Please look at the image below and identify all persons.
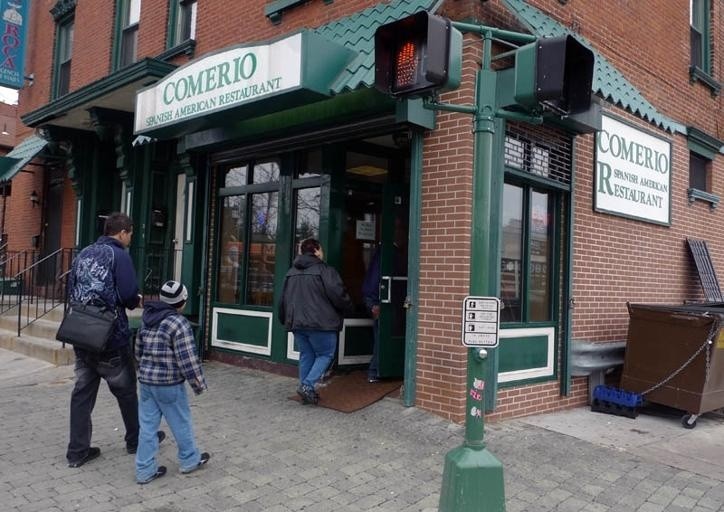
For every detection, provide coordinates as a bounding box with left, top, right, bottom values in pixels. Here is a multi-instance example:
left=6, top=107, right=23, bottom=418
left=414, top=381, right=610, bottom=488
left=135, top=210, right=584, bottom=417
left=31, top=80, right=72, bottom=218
left=133, top=279, right=212, bottom=483
left=360, top=225, right=381, bottom=384
left=63, top=212, right=166, bottom=468
left=276, top=236, right=356, bottom=406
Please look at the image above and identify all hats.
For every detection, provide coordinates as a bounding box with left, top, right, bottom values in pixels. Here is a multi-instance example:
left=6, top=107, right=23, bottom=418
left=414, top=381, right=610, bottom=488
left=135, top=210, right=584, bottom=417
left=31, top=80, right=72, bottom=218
left=160, top=280, right=189, bottom=309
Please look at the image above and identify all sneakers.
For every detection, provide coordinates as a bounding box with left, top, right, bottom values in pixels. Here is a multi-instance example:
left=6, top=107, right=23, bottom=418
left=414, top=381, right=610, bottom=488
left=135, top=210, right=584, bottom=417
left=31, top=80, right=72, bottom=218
left=67, top=447, right=100, bottom=467
left=137, top=466, right=165, bottom=484
left=296, top=383, right=320, bottom=405
left=128, top=430, right=165, bottom=453
left=368, top=376, right=382, bottom=383
left=182, top=452, right=210, bottom=474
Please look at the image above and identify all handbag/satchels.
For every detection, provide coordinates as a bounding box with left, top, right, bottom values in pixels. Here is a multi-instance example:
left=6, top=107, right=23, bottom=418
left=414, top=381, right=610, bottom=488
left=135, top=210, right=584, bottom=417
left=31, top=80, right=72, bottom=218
left=56, top=302, right=117, bottom=355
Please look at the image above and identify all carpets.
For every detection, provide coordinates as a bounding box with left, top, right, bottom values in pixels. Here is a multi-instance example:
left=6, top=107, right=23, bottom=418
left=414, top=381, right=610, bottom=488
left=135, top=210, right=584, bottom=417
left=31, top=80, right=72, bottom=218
left=289, top=370, right=404, bottom=414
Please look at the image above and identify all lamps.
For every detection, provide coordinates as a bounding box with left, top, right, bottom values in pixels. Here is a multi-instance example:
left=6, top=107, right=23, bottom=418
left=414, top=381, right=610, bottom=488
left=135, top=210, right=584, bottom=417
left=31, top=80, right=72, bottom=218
left=30, top=190, right=39, bottom=208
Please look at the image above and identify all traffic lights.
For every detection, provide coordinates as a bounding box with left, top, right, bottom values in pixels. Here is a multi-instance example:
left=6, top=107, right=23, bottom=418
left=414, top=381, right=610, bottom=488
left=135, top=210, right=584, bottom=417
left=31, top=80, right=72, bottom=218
left=371, top=10, right=451, bottom=100
left=535, top=30, right=597, bottom=115
